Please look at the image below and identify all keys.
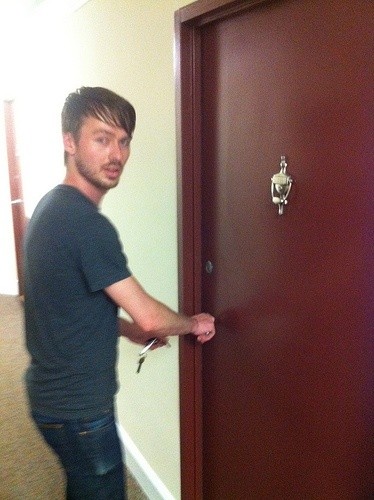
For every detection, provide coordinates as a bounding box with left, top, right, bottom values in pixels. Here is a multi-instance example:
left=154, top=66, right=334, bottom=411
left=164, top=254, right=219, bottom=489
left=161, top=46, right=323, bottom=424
left=135, top=351, right=148, bottom=374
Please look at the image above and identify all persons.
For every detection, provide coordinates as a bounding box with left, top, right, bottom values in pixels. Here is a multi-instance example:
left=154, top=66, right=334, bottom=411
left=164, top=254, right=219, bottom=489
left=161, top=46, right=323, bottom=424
left=22, top=86, right=216, bottom=499
